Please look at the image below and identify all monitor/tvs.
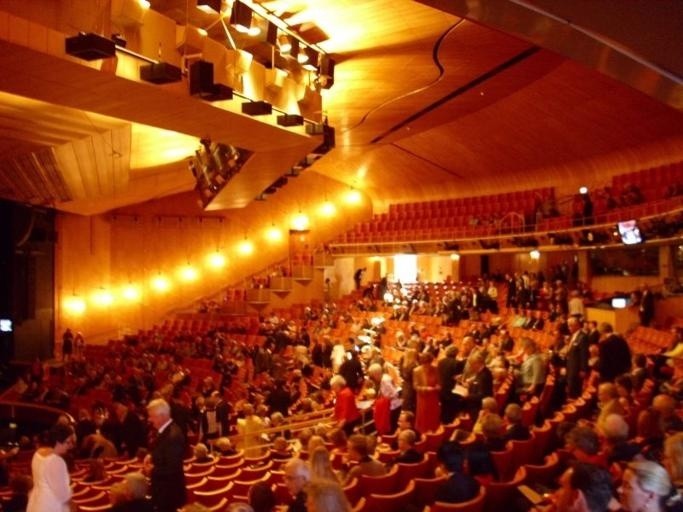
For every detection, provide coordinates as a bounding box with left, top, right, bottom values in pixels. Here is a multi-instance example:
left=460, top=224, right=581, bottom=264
left=617, top=219, right=644, bottom=246
left=611, top=298, right=626, bottom=309
left=0, top=319, right=14, bottom=333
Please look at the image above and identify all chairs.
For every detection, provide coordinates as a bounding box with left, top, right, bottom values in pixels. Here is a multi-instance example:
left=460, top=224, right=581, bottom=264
left=0, top=157, right=683, bottom=512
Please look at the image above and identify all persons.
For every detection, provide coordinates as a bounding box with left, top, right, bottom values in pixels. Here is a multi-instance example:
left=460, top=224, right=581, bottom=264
left=1, top=180, right=682, bottom=512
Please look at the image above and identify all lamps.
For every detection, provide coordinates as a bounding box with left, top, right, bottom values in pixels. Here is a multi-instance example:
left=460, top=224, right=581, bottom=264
left=193, top=0, right=337, bottom=91
left=61, top=33, right=332, bottom=143
left=254, top=123, right=336, bottom=205
left=181, top=136, right=243, bottom=199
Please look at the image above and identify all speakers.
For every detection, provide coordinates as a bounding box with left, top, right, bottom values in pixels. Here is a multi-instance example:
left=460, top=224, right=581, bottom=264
left=189, top=61, right=214, bottom=98
